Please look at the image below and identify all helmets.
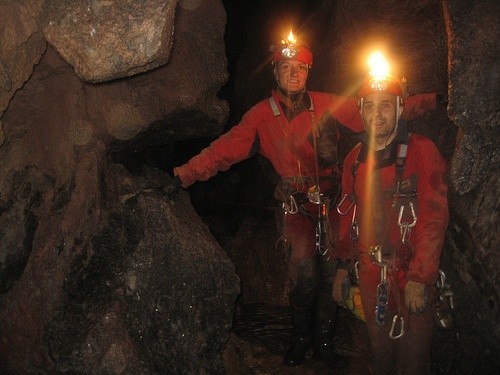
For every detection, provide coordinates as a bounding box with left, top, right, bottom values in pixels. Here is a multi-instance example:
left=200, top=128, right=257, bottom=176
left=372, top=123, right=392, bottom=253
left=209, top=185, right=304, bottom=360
left=357, top=75, right=403, bottom=100
left=273, top=40, right=313, bottom=68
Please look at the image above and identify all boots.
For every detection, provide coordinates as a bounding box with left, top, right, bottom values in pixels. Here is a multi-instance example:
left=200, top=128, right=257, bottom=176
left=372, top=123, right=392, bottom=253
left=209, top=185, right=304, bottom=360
left=282, top=288, right=312, bottom=367
left=314, top=283, right=340, bottom=374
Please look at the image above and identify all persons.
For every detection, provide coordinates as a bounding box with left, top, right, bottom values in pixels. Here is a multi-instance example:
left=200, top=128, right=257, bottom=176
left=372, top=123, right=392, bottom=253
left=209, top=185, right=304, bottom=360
left=332, top=79, right=449, bottom=373
left=147, top=42, right=446, bottom=368
